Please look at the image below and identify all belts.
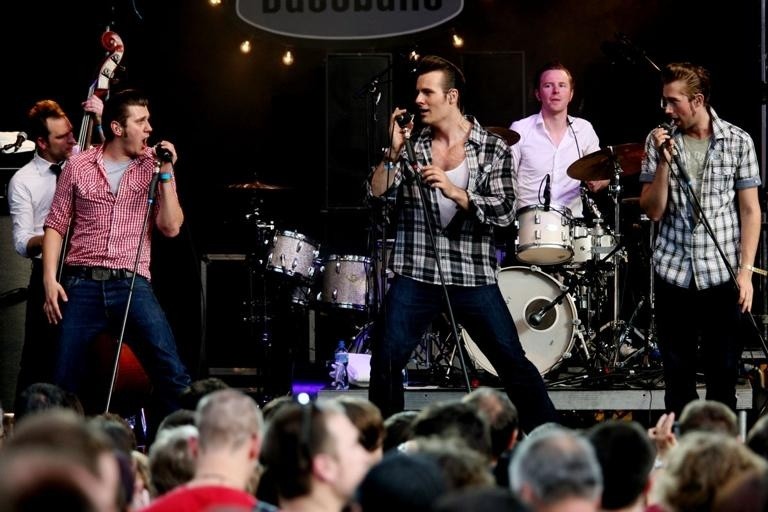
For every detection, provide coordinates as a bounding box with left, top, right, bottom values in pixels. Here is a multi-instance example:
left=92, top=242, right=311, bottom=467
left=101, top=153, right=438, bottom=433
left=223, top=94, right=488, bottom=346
left=65, top=266, right=133, bottom=281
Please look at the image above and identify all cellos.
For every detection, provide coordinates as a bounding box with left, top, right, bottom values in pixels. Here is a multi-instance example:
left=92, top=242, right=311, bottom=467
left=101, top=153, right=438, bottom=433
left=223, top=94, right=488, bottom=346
left=77, top=26, right=150, bottom=397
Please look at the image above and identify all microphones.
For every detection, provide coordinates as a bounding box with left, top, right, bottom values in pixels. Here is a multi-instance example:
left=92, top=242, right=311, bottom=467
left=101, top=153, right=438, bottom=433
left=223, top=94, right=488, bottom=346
left=14, top=131, right=27, bottom=152
left=152, top=142, right=172, bottom=162
left=657, top=118, right=681, bottom=152
left=395, top=112, right=411, bottom=128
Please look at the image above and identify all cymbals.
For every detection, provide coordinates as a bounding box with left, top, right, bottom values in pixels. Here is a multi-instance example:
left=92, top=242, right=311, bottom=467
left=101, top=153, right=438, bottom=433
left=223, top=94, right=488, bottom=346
left=567, top=142, right=648, bottom=181
left=622, top=197, right=644, bottom=203
left=226, top=181, right=281, bottom=192
left=317, top=205, right=371, bottom=211
left=483, top=126, right=520, bottom=147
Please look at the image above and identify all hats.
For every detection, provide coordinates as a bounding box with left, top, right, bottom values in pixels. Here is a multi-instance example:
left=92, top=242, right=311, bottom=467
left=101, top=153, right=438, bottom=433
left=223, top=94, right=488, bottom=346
left=423, top=45, right=466, bottom=83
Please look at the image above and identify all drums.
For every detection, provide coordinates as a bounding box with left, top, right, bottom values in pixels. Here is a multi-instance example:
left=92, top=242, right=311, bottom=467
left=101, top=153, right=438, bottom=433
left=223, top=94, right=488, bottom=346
left=515, top=205, right=575, bottom=265
left=316, top=255, right=377, bottom=312
left=292, top=286, right=311, bottom=305
left=265, top=230, right=322, bottom=281
left=461, top=267, right=581, bottom=377
left=562, top=227, right=618, bottom=277
left=377, top=238, right=397, bottom=277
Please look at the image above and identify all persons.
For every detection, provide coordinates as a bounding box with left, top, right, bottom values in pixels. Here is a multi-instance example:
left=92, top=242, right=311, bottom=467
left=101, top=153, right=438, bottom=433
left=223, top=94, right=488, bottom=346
left=178, top=379, right=229, bottom=412
left=379, top=411, right=422, bottom=457
left=146, top=426, right=202, bottom=500
left=9, top=95, right=107, bottom=430
left=40, top=87, right=190, bottom=414
left=591, top=420, right=654, bottom=512
left=409, top=398, right=498, bottom=473
left=140, top=388, right=280, bottom=512
left=675, top=400, right=742, bottom=449
left=0, top=406, right=120, bottom=511
left=509, top=65, right=609, bottom=221
left=509, top=423, right=603, bottom=512
left=463, top=388, right=519, bottom=468
left=10, top=381, right=84, bottom=434
left=641, top=62, right=763, bottom=424
left=261, top=399, right=367, bottom=512
left=333, top=395, right=384, bottom=465
left=365, top=57, right=562, bottom=439
left=353, top=438, right=497, bottom=512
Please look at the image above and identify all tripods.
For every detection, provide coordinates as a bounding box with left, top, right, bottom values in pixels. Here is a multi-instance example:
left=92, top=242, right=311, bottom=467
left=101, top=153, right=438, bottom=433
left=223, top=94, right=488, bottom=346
left=421, top=316, right=471, bottom=387
left=578, top=185, right=661, bottom=387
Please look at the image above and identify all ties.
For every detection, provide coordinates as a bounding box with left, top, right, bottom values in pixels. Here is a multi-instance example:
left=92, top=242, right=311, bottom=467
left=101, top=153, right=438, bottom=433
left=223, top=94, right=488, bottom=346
left=49, top=162, right=63, bottom=186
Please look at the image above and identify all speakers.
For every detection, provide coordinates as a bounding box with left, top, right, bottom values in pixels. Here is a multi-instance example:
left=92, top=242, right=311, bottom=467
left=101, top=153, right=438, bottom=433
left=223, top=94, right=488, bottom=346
left=623, top=198, right=660, bottom=268
